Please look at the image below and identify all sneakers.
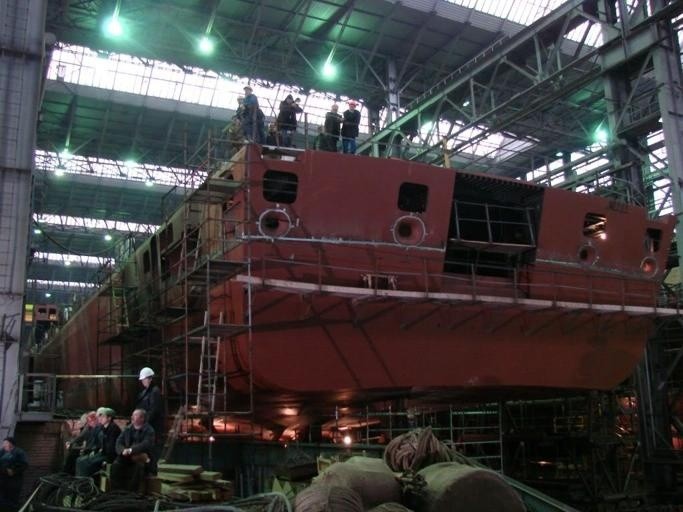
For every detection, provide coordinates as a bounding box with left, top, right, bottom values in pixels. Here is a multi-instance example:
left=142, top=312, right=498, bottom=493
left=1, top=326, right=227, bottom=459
left=40, top=476, right=64, bottom=486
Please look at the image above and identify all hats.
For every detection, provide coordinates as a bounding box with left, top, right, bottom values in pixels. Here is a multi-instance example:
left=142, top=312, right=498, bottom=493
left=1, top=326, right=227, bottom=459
left=287, top=95, right=293, bottom=99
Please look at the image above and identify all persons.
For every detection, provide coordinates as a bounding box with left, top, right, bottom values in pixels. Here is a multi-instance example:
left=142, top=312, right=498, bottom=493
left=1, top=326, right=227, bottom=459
left=0, top=437, right=28, bottom=512
left=222, top=86, right=303, bottom=147
left=65, top=366, right=165, bottom=492
left=341, top=101, right=361, bottom=154
left=324, top=105, right=344, bottom=152
left=312, top=124, right=328, bottom=152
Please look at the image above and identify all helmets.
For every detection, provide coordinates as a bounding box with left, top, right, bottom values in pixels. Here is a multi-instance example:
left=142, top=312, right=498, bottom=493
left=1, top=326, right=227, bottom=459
left=237, top=86, right=253, bottom=100
left=80, top=407, right=115, bottom=425
left=139, top=367, right=155, bottom=381
left=349, top=102, right=356, bottom=107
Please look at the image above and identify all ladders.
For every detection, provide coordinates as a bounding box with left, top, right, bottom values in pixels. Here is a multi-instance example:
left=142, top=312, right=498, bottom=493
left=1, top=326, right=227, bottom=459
left=111, top=280, right=129, bottom=335
left=177, top=173, right=209, bottom=284
left=161, top=406, right=186, bottom=463
left=196, top=310, right=223, bottom=413
